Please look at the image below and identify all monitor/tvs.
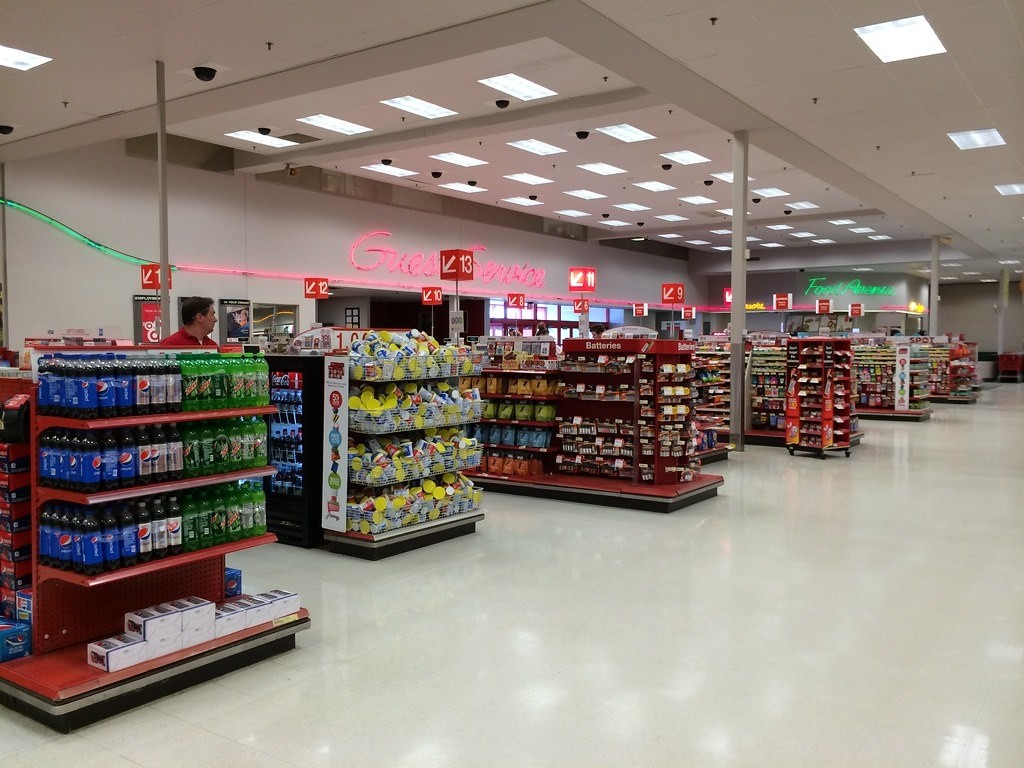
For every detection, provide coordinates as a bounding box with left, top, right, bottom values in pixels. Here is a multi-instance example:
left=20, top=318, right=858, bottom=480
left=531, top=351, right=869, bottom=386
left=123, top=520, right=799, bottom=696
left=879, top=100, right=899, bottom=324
left=241, top=343, right=260, bottom=354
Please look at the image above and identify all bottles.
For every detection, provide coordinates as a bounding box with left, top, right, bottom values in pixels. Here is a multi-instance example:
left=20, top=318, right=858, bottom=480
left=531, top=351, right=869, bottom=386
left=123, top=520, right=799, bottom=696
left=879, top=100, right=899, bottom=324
left=37, top=353, right=268, bottom=575
left=329, top=423, right=342, bottom=447
left=271, top=465, right=303, bottom=494
left=271, top=372, right=289, bottom=387
left=328, top=496, right=340, bottom=511
left=328, top=472, right=342, bottom=496
left=271, top=389, right=302, bottom=413
left=271, top=427, right=303, bottom=464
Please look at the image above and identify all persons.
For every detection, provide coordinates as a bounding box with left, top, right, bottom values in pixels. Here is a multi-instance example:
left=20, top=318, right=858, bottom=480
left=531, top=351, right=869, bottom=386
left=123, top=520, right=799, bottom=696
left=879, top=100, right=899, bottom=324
left=161, top=296, right=221, bottom=353
left=534, top=321, right=561, bottom=358
left=509, top=328, right=522, bottom=338
left=590, top=324, right=610, bottom=340
left=234, top=310, right=248, bottom=327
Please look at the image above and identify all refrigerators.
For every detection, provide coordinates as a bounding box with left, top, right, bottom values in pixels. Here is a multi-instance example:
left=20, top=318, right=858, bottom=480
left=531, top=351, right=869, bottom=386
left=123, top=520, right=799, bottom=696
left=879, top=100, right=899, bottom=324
left=263, top=355, right=325, bottom=549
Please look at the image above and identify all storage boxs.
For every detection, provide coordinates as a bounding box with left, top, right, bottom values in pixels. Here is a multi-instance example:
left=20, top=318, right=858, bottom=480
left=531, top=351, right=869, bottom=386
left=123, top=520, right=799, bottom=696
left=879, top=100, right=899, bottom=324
left=909, top=400, right=930, bottom=410
left=781, top=338, right=787, bottom=345
left=910, top=375, right=929, bottom=382
left=785, top=314, right=804, bottom=331
left=752, top=333, right=777, bottom=345
left=714, top=323, right=731, bottom=336
left=835, top=314, right=854, bottom=332
left=819, top=314, right=837, bottom=332
left=910, top=362, right=929, bottom=370
left=803, top=314, right=820, bottom=331
left=0, top=443, right=33, bottom=665
left=86, top=567, right=301, bottom=674
left=602, top=331, right=658, bottom=339
left=473, top=341, right=562, bottom=371
left=911, top=350, right=929, bottom=358
left=914, top=386, right=929, bottom=396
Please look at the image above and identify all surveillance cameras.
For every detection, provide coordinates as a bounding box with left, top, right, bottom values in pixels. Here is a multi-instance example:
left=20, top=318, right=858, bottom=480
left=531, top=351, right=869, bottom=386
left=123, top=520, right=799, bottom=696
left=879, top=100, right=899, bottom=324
left=704, top=180, right=713, bottom=186
left=496, top=100, right=509, bottom=108
left=192, top=67, right=217, bottom=81
left=752, top=198, right=761, bottom=203
left=662, top=164, right=672, bottom=170
left=431, top=172, right=442, bottom=178
left=258, top=127, right=271, bottom=135
left=381, top=159, right=392, bottom=165
left=0, top=125, right=14, bottom=135
left=468, top=180, right=477, bottom=186
left=784, top=210, right=792, bottom=215
left=798, top=269, right=805, bottom=272
left=576, top=131, right=590, bottom=139
left=637, top=222, right=644, bottom=226
left=602, top=214, right=609, bottom=218
left=529, top=196, right=537, bottom=200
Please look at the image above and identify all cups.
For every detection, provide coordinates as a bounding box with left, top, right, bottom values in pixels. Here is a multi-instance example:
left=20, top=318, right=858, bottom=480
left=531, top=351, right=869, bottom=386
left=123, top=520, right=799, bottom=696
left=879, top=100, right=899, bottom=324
left=345, top=329, right=481, bottom=533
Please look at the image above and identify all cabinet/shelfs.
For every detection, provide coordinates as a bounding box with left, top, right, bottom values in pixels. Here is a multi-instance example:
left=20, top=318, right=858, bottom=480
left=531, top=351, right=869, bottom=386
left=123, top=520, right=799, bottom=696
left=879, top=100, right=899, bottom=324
left=695, top=333, right=752, bottom=446
left=0, top=346, right=311, bottom=738
left=459, top=355, right=565, bottom=499
left=694, top=364, right=735, bottom=464
left=533, top=338, right=725, bottom=515
left=929, top=343, right=979, bottom=405
left=852, top=343, right=934, bottom=422
left=786, top=339, right=852, bottom=461
left=850, top=378, right=865, bottom=446
left=323, top=344, right=488, bottom=562
left=743, top=337, right=787, bottom=450
